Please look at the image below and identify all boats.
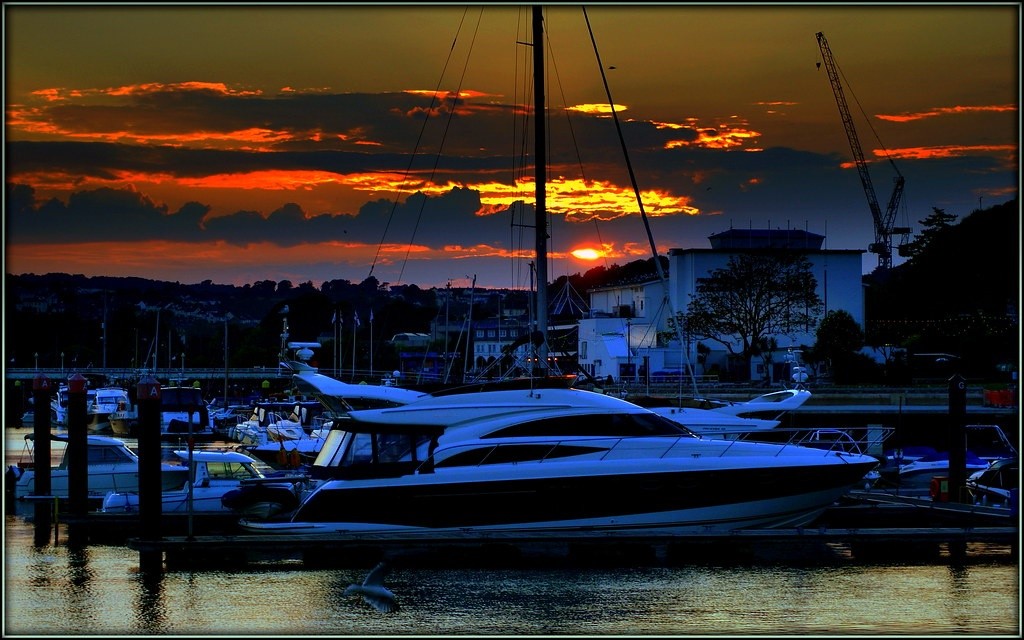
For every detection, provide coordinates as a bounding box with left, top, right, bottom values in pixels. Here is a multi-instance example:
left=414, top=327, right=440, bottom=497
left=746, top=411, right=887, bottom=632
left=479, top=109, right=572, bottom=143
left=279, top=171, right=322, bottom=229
left=0, top=6, right=1018, bottom=572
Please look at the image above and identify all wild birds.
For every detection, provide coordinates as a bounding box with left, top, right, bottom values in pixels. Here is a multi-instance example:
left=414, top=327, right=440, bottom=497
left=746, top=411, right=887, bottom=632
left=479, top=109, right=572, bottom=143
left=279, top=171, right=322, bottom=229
left=344, top=562, right=403, bottom=613
